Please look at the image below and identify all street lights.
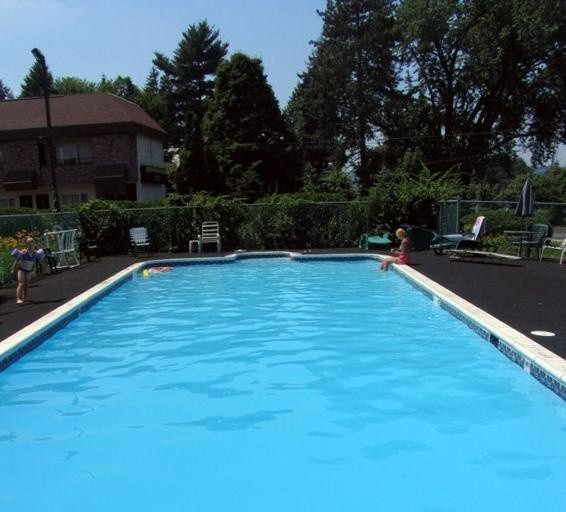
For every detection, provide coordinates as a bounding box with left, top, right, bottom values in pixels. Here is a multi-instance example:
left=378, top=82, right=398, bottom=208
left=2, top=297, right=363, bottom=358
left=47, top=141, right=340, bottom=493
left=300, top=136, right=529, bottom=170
left=28, top=48, right=61, bottom=219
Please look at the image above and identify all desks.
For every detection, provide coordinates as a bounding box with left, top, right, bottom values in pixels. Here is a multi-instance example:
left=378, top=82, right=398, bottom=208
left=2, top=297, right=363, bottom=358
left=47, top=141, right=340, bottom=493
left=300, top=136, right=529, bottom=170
left=503, top=229, right=537, bottom=261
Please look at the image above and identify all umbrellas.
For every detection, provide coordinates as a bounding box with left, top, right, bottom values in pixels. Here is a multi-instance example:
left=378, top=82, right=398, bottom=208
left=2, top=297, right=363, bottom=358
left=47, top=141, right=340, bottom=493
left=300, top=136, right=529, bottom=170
left=514, top=177, right=535, bottom=256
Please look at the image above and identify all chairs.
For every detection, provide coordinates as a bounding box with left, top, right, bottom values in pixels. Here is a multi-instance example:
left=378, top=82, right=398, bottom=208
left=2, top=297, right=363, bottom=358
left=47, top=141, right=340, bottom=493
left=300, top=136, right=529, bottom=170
left=429, top=216, right=486, bottom=256
left=35, top=247, right=58, bottom=274
left=359, top=232, right=393, bottom=250
left=539, top=237, right=566, bottom=264
left=198, top=221, right=222, bottom=255
left=513, top=223, right=553, bottom=261
left=129, top=227, right=152, bottom=258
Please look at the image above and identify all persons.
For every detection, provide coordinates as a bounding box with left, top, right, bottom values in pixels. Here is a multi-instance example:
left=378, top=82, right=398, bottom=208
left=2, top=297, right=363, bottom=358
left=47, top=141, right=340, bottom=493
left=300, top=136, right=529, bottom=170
left=141, top=264, right=174, bottom=278
left=10, top=236, right=46, bottom=304
left=381, top=227, right=412, bottom=272
